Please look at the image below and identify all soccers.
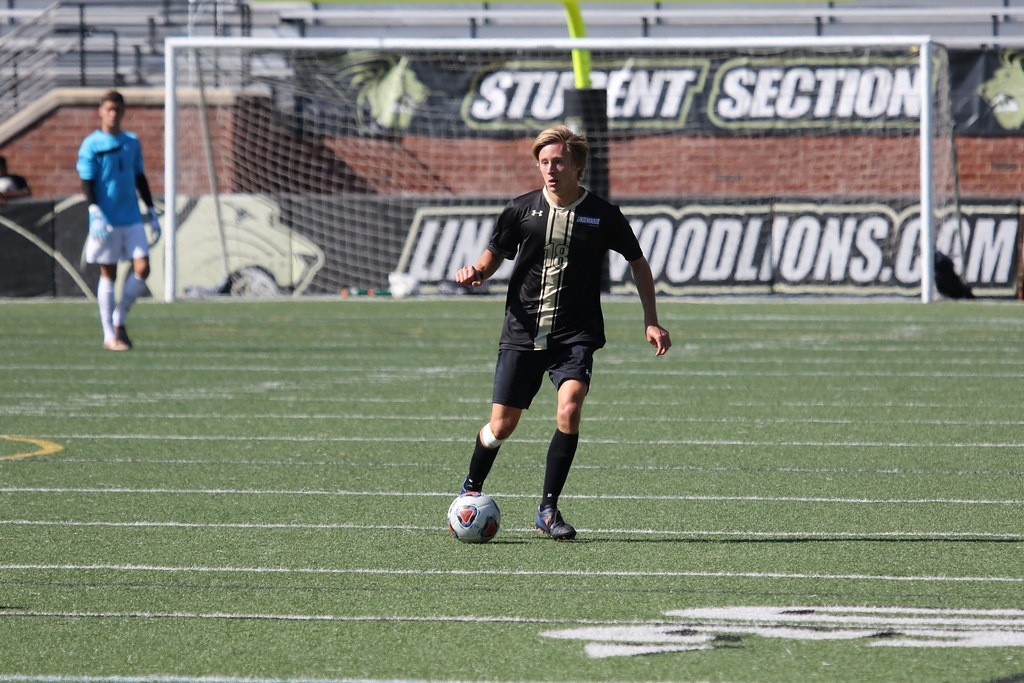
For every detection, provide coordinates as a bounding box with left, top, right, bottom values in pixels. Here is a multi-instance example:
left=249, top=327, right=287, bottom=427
left=449, top=489, right=505, bottom=543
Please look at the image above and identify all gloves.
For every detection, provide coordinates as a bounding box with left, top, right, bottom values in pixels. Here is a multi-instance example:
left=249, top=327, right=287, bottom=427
left=88, top=204, right=113, bottom=245
left=148, top=207, right=161, bottom=246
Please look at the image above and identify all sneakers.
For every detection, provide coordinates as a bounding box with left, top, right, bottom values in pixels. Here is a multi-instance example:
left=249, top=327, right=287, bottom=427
left=535, top=505, right=576, bottom=541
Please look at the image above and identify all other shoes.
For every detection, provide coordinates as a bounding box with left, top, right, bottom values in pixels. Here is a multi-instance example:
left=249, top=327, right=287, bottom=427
left=103, top=337, right=129, bottom=350
left=114, top=325, right=132, bottom=348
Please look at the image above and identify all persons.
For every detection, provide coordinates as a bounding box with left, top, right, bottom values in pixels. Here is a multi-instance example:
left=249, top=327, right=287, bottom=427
left=76, top=90, right=162, bottom=351
left=457, top=123, right=672, bottom=540
left=0, top=155, right=33, bottom=204
